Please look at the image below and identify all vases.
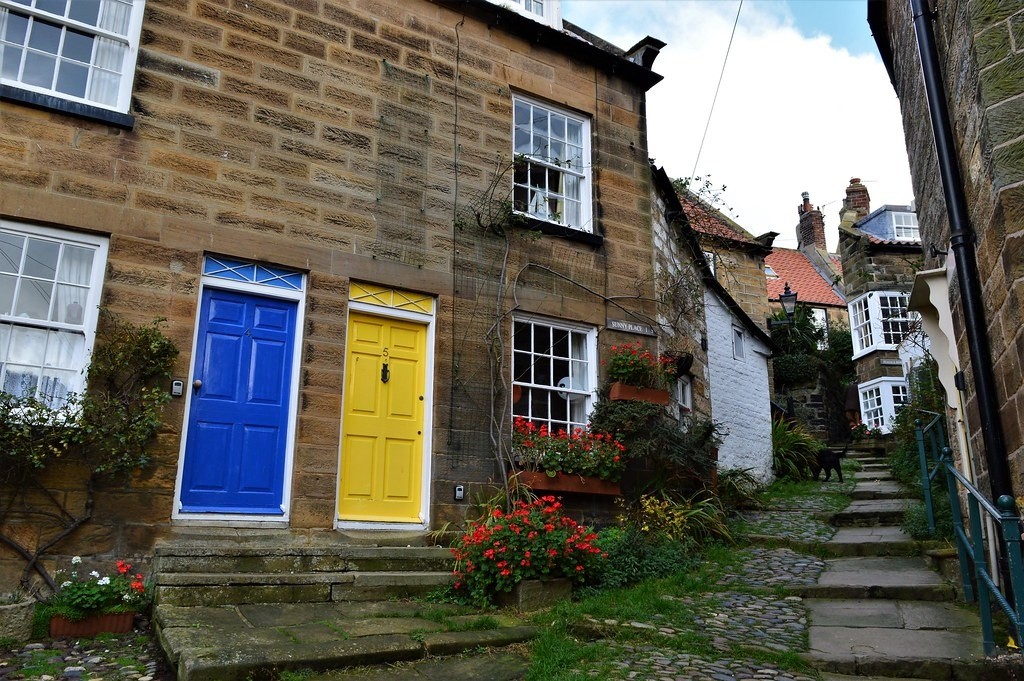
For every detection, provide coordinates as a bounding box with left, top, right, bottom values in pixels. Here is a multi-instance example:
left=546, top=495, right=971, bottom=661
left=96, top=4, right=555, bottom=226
left=48, top=607, right=136, bottom=640
left=606, top=379, right=670, bottom=406
left=503, top=576, right=574, bottom=613
left=507, top=468, right=621, bottom=496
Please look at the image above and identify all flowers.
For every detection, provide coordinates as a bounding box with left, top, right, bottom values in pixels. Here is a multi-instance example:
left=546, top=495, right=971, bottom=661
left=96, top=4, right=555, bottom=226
left=450, top=487, right=605, bottom=596
left=511, top=413, right=624, bottom=468
left=601, top=336, right=681, bottom=382
left=55, top=553, right=146, bottom=608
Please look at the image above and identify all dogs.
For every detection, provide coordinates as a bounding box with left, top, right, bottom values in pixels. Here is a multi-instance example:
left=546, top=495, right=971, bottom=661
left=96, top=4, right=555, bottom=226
left=810, top=443, right=847, bottom=482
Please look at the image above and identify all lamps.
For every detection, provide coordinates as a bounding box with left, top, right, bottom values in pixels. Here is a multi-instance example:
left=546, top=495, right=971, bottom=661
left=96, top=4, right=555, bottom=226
left=767, top=282, right=798, bottom=330
left=930, top=242, right=948, bottom=258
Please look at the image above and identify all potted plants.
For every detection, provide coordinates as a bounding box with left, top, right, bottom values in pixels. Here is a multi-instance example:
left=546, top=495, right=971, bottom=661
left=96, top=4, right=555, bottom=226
left=664, top=331, right=695, bottom=380
